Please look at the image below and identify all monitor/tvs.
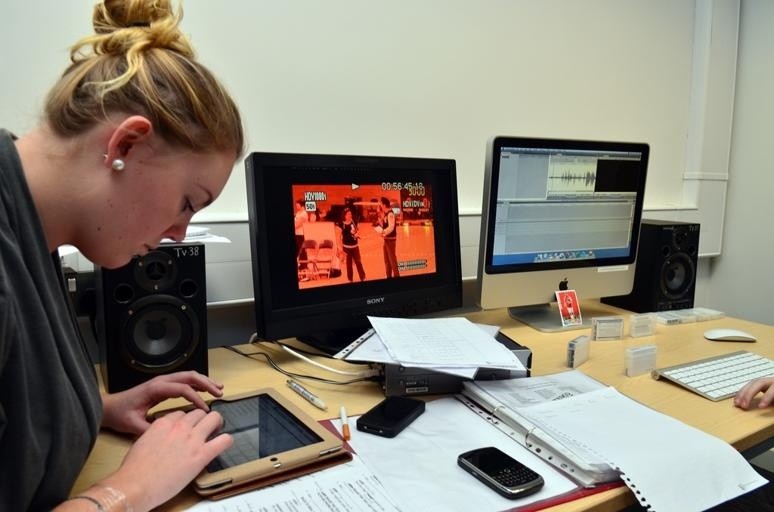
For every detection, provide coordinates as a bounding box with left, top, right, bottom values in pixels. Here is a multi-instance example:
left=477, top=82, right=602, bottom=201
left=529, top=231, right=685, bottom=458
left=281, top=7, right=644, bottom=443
left=474, top=132, right=651, bottom=334
left=243, top=151, right=464, bottom=365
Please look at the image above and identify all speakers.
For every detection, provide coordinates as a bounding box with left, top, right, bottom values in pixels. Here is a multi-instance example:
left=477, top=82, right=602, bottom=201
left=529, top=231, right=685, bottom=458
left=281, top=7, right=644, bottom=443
left=600, top=219, right=701, bottom=313
left=101, top=242, right=209, bottom=396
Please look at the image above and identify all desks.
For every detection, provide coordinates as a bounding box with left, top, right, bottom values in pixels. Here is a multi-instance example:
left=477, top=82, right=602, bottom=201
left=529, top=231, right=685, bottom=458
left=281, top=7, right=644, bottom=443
left=67, top=294, right=774, bottom=512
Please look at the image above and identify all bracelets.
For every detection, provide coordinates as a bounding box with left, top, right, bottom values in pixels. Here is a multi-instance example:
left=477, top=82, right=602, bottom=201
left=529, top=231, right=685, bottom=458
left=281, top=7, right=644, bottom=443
left=71, top=491, right=102, bottom=511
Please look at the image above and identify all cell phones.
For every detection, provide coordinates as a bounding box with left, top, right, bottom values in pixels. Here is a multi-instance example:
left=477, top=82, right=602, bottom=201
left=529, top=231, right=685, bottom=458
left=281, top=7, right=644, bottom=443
left=354, top=393, right=426, bottom=437
left=458, top=445, right=545, bottom=499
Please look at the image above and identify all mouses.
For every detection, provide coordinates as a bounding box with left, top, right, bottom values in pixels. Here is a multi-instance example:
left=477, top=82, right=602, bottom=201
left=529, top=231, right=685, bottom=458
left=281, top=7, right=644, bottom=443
left=703, top=328, right=755, bottom=344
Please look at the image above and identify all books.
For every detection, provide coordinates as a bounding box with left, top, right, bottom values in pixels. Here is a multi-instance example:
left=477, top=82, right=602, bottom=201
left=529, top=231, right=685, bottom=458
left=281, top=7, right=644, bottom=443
left=319, top=370, right=627, bottom=512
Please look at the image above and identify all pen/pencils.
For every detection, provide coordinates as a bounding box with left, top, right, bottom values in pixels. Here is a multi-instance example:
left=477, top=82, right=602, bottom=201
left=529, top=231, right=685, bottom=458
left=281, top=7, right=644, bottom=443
left=286, top=377, right=328, bottom=411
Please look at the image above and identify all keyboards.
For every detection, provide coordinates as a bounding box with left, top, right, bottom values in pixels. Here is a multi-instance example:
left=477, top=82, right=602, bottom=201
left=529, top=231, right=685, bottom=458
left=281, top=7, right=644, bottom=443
left=652, top=350, right=774, bottom=404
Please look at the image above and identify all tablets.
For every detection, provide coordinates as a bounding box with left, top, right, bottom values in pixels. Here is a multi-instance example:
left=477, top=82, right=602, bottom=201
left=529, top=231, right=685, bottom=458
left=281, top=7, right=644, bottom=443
left=151, top=386, right=353, bottom=501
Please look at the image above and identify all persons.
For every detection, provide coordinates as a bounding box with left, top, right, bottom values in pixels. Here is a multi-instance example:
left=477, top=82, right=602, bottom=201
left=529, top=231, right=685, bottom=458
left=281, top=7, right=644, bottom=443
left=336, top=207, right=366, bottom=281
left=734, top=374, right=773, bottom=411
left=562, top=292, right=575, bottom=325
left=291, top=199, right=310, bottom=271
left=1, top=0, right=243, bottom=510
left=377, top=197, right=400, bottom=279
